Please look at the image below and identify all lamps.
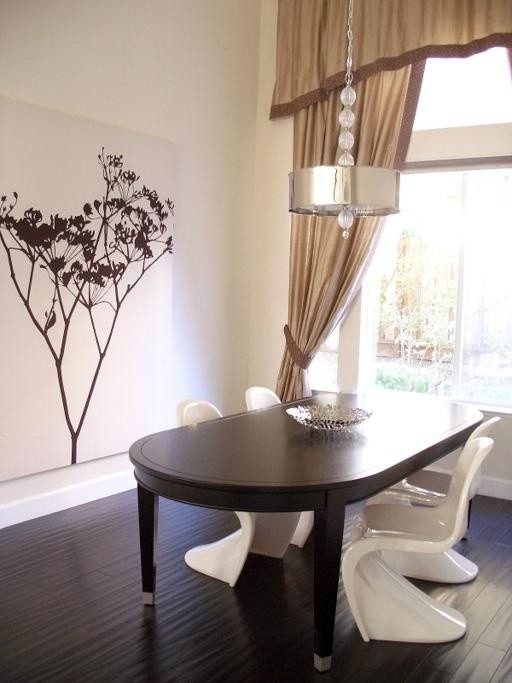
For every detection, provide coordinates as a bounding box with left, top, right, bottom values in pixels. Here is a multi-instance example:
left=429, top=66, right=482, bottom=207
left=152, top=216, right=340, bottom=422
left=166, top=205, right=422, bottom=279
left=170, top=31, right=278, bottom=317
left=175, top=387, right=320, bottom=589
left=341, top=415, right=502, bottom=641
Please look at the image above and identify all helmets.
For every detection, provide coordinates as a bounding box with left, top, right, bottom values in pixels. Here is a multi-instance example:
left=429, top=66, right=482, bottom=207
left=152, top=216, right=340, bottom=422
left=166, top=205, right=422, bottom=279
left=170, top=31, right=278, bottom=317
left=286, top=403, right=372, bottom=432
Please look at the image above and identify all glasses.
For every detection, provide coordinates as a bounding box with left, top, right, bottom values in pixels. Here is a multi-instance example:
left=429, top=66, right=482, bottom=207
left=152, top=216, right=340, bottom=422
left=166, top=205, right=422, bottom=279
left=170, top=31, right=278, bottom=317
left=288, top=0, right=403, bottom=242
